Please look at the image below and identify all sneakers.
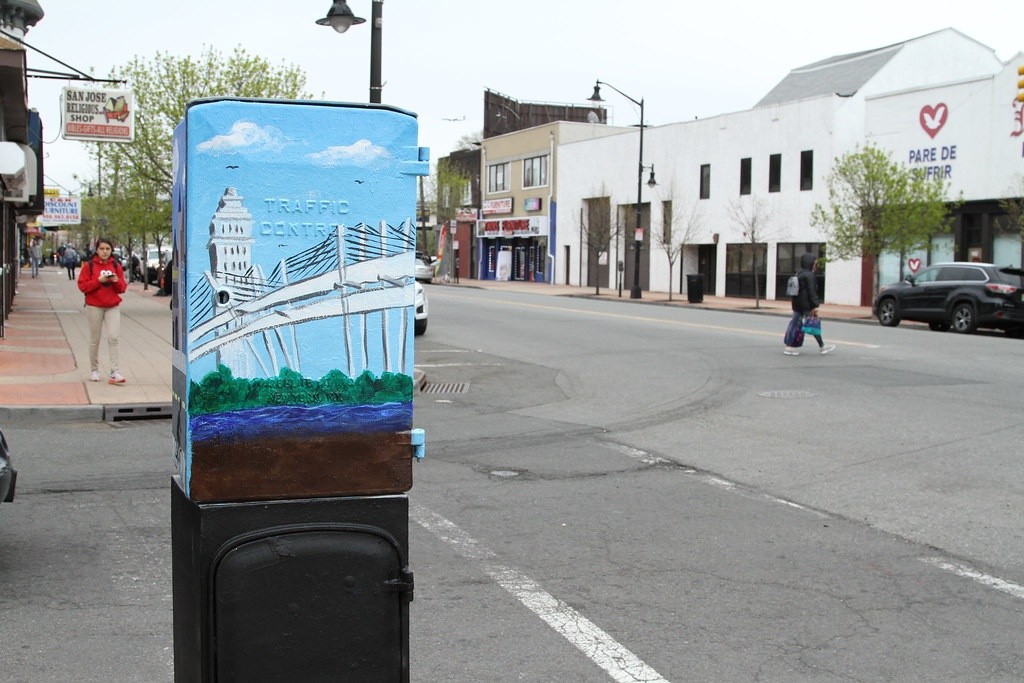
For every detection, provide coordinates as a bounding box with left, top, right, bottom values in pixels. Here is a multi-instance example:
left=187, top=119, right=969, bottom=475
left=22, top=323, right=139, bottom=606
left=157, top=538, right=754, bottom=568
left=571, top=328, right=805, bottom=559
left=90, top=371, right=99, bottom=381
left=109, top=372, right=125, bottom=383
left=820, top=344, right=836, bottom=353
left=783, top=348, right=800, bottom=355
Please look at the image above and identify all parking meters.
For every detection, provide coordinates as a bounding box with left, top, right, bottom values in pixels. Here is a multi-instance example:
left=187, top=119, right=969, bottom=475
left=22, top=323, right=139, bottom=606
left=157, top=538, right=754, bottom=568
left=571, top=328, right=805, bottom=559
left=618, top=261, right=623, bottom=297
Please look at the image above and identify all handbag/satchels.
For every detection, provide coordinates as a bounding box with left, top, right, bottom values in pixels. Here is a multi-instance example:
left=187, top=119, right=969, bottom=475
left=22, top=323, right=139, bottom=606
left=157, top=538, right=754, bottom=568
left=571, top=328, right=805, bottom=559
left=802, top=310, right=820, bottom=335
left=783, top=312, right=804, bottom=347
left=787, top=270, right=800, bottom=296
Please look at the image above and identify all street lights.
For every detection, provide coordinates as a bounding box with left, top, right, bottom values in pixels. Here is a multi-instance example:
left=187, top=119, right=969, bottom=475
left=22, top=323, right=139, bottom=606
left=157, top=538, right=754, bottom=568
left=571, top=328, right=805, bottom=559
left=586, top=80, right=659, bottom=299
left=315, top=0, right=384, bottom=104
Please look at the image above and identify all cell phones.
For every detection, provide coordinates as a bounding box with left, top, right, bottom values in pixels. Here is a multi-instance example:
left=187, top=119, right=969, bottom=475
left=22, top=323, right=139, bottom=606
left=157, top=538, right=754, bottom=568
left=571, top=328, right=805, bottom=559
left=106, top=274, right=115, bottom=278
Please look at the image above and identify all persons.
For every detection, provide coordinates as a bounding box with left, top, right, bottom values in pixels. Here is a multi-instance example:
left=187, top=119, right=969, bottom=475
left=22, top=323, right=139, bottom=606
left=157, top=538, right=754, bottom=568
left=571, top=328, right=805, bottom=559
left=421, top=250, right=430, bottom=263
left=784, top=254, right=835, bottom=355
left=78, top=237, right=126, bottom=385
left=24, top=240, right=92, bottom=279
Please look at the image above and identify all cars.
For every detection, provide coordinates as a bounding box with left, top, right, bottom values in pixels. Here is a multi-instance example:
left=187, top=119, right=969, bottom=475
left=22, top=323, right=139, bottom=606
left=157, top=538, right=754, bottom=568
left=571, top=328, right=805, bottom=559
left=414, top=281, right=429, bottom=334
left=872, top=262, right=1024, bottom=339
left=415, top=251, right=433, bottom=284
left=138, top=249, right=162, bottom=284
left=114, top=249, right=127, bottom=270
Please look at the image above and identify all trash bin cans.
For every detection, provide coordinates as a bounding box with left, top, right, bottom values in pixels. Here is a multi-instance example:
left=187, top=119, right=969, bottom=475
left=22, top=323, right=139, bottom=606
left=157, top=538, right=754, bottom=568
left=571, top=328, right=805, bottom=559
left=687, top=274, right=703, bottom=303
